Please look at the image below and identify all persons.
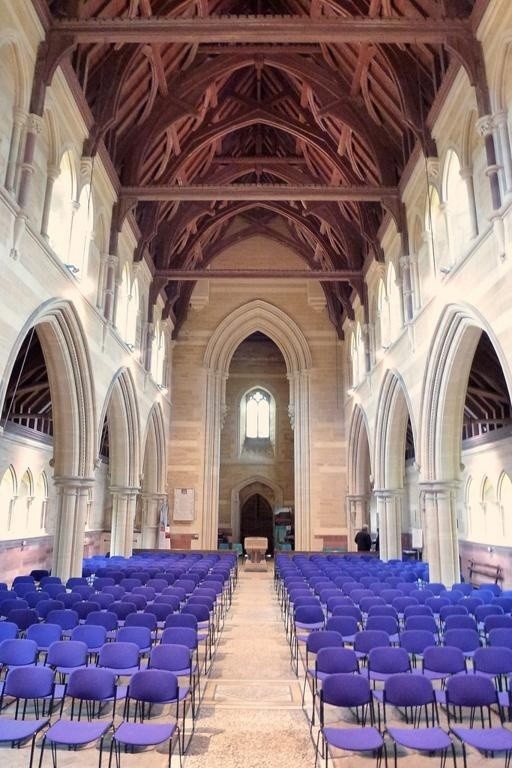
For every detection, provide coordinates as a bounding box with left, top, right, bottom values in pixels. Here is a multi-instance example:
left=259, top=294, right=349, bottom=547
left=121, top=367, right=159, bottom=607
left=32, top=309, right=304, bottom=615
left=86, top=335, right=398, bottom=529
left=371, top=528, right=379, bottom=554
left=353, top=524, right=372, bottom=555
